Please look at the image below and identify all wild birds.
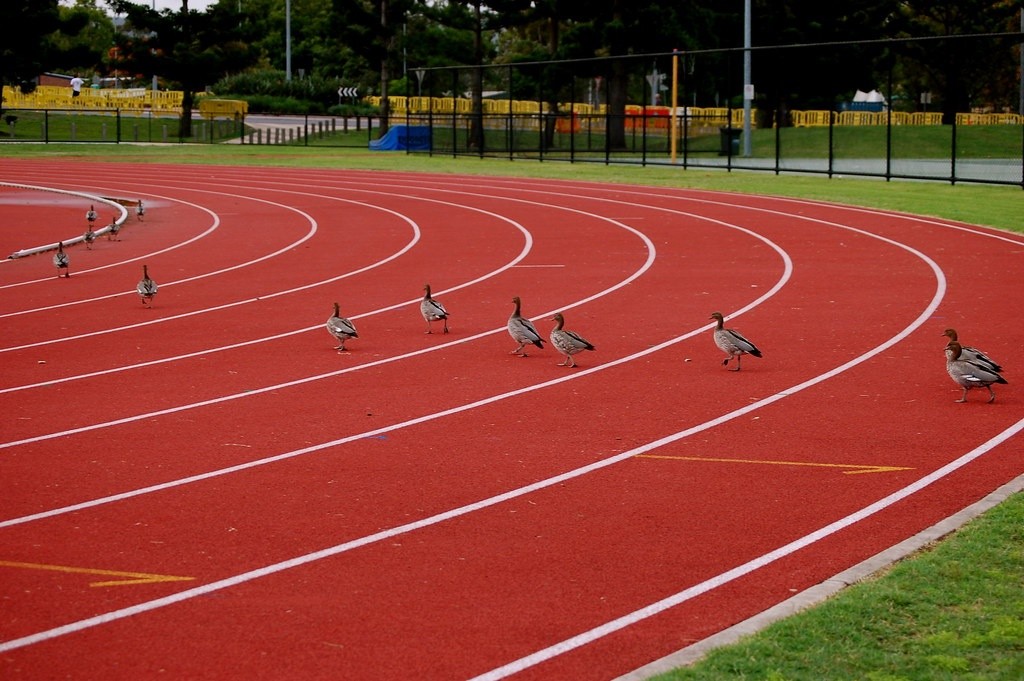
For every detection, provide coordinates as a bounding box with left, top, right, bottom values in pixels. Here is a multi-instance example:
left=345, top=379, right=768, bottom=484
left=941, top=328, right=1008, bottom=404
left=506, top=295, right=598, bottom=367
left=326, top=302, right=360, bottom=351
left=53, top=200, right=159, bottom=309
left=708, top=311, right=764, bottom=371
left=419, top=283, right=451, bottom=334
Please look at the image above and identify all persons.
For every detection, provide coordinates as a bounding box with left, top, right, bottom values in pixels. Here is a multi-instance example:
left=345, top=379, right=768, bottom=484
left=70, top=74, right=85, bottom=96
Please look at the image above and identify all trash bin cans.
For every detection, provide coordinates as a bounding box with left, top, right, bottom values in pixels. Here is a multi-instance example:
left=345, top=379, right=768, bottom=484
left=719, top=128, right=743, bottom=155
